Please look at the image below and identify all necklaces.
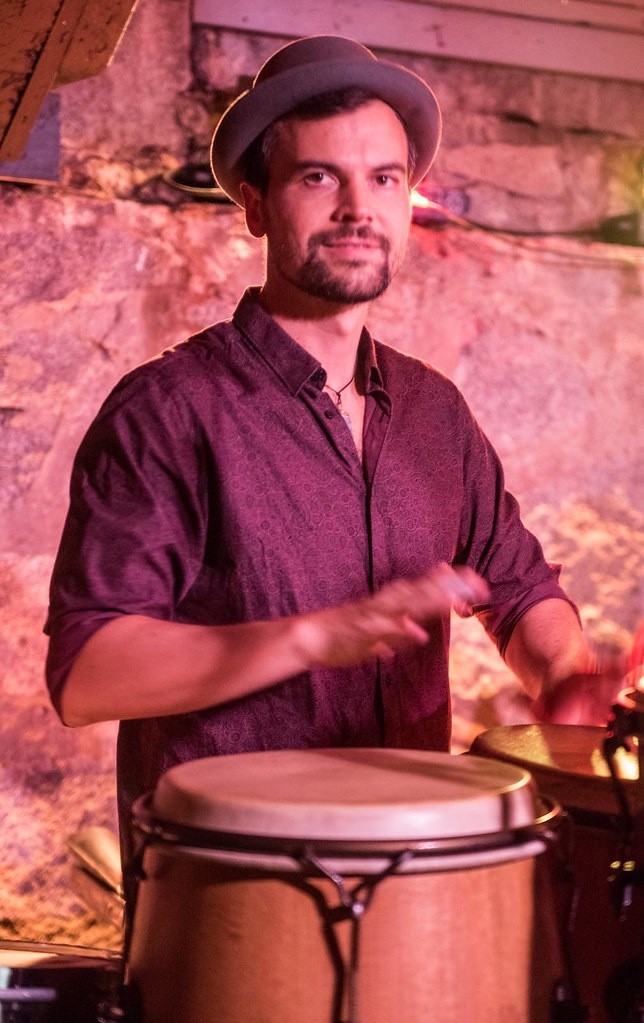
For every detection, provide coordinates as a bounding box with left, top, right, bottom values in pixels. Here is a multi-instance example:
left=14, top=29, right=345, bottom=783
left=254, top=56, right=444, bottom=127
left=324, top=373, right=356, bottom=438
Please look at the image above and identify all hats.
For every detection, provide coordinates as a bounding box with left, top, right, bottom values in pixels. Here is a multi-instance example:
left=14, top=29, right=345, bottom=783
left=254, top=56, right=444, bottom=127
left=210, top=36, right=442, bottom=212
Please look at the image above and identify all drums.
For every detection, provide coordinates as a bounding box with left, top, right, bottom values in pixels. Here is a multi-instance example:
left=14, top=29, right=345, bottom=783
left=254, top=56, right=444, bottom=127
left=468, top=724, right=644, bottom=1023
left=119, top=746, right=569, bottom=1023
left=0, top=940, right=124, bottom=1023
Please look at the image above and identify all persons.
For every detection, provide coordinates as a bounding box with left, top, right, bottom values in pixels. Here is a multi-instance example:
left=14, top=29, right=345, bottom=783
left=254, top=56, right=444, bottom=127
left=41, top=34, right=634, bottom=956
